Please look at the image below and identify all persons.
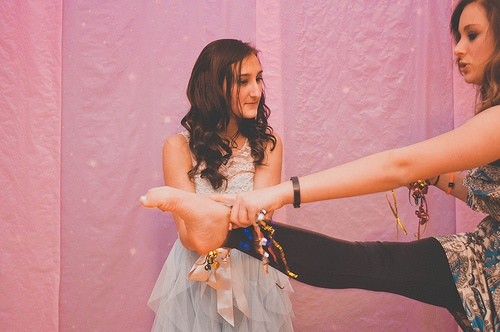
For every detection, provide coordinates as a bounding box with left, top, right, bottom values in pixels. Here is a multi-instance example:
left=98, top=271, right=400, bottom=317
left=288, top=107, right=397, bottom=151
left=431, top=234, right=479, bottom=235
left=147, top=39, right=295, bottom=332
left=140, top=0, right=500, bottom=332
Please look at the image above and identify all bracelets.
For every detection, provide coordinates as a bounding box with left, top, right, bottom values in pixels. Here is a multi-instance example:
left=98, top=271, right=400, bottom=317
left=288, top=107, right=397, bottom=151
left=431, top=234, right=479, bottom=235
left=447, top=172, right=454, bottom=194
left=434, top=176, right=439, bottom=185
left=290, top=176, right=300, bottom=208
